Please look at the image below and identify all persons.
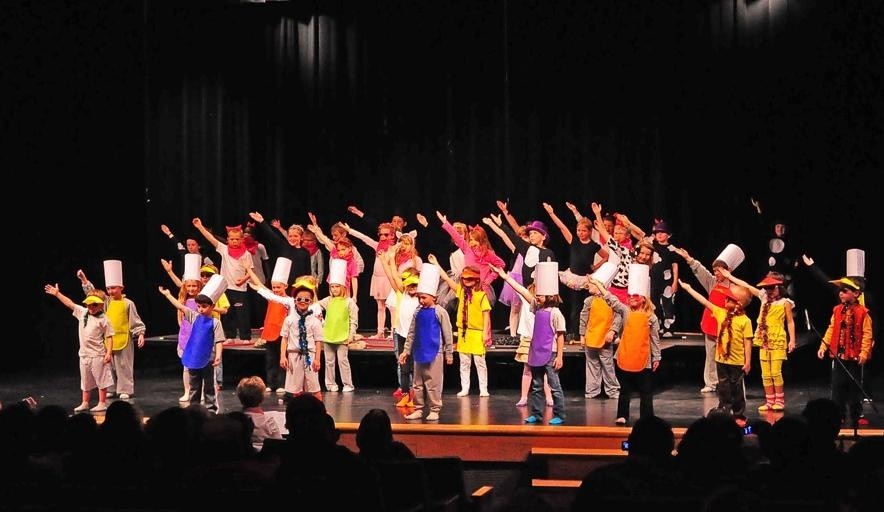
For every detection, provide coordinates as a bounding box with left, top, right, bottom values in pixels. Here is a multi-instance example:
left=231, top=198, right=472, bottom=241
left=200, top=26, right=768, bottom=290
left=144, top=191, right=674, bottom=394
left=41, top=281, right=114, bottom=412
left=0, top=388, right=882, bottom=510
left=278, top=285, right=322, bottom=401
left=487, top=262, right=569, bottom=425
left=585, top=262, right=660, bottom=424
left=155, top=284, right=224, bottom=414
left=716, top=263, right=795, bottom=413
left=391, top=290, right=453, bottom=423
left=674, top=241, right=745, bottom=393
left=813, top=274, right=873, bottom=423
left=678, top=276, right=755, bottom=415
left=74, top=269, right=149, bottom=401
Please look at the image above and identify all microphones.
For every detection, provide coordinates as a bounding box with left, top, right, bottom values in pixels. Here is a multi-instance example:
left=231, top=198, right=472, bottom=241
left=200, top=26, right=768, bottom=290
left=805, top=309, right=811, bottom=330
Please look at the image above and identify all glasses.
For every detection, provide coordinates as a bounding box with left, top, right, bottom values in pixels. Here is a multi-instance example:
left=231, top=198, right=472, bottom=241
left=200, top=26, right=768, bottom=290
left=837, top=287, right=852, bottom=294
left=296, top=297, right=313, bottom=304
left=763, top=285, right=777, bottom=290
left=85, top=304, right=98, bottom=307
left=379, top=233, right=389, bottom=237
left=627, top=294, right=641, bottom=301
left=726, top=297, right=732, bottom=303
left=201, top=272, right=212, bottom=277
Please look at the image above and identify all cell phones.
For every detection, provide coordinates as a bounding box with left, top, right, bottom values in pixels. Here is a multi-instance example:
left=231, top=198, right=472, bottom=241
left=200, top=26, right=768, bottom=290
left=743, top=425, right=752, bottom=435
left=622, top=441, right=629, bottom=450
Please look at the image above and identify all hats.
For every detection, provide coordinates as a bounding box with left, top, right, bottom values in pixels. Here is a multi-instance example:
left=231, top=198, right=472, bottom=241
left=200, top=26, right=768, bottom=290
left=525, top=221, right=549, bottom=240
left=200, top=266, right=215, bottom=273
left=198, top=274, right=228, bottom=304
left=103, top=260, right=123, bottom=288
left=460, top=271, right=480, bottom=279
left=271, top=256, right=292, bottom=285
left=715, top=243, right=746, bottom=271
left=184, top=254, right=202, bottom=281
left=592, top=262, right=619, bottom=289
left=329, top=258, right=347, bottom=286
left=627, top=264, right=649, bottom=296
left=828, top=277, right=860, bottom=290
left=399, top=272, right=411, bottom=279
left=416, top=263, right=441, bottom=297
left=723, top=285, right=752, bottom=309
left=293, top=282, right=317, bottom=289
left=401, top=275, right=419, bottom=287
left=82, top=296, right=104, bottom=305
left=756, top=276, right=783, bottom=287
left=535, top=261, right=558, bottom=296
